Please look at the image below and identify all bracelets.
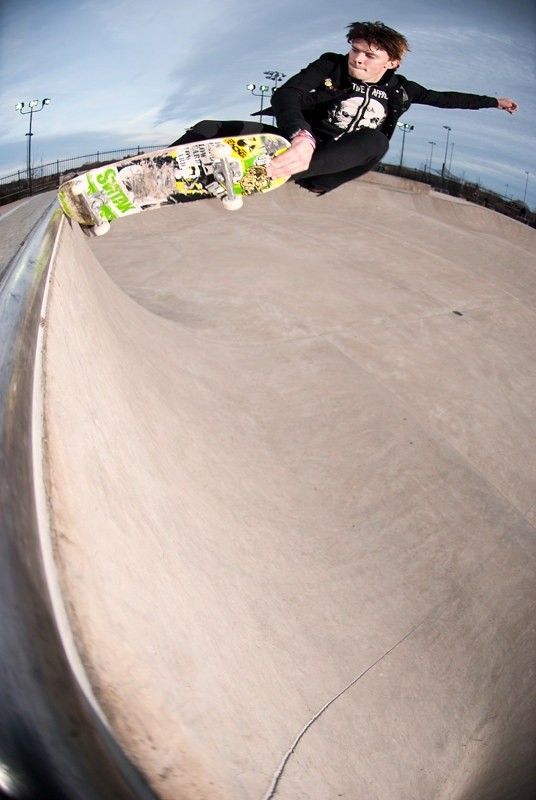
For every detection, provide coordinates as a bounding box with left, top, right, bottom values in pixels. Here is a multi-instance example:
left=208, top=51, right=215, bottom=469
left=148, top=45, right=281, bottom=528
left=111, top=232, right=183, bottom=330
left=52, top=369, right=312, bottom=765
left=290, top=129, right=316, bottom=150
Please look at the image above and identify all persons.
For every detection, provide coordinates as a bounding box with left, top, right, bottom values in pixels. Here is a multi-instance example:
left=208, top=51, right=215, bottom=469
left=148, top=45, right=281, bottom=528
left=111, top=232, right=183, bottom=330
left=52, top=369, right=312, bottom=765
left=168, top=21, right=519, bottom=197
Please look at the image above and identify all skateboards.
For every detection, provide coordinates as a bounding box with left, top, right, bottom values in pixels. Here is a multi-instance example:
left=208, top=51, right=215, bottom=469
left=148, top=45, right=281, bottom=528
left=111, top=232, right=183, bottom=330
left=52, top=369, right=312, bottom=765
left=56, top=131, right=293, bottom=237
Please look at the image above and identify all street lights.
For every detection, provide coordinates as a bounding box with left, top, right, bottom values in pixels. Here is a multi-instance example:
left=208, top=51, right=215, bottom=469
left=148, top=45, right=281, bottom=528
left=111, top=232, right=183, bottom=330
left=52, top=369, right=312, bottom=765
left=264, top=69, right=287, bottom=127
left=247, top=83, right=277, bottom=123
left=14, top=97, right=52, bottom=196
left=397, top=121, right=415, bottom=176
left=426, top=141, right=436, bottom=184
left=440, top=125, right=452, bottom=193
